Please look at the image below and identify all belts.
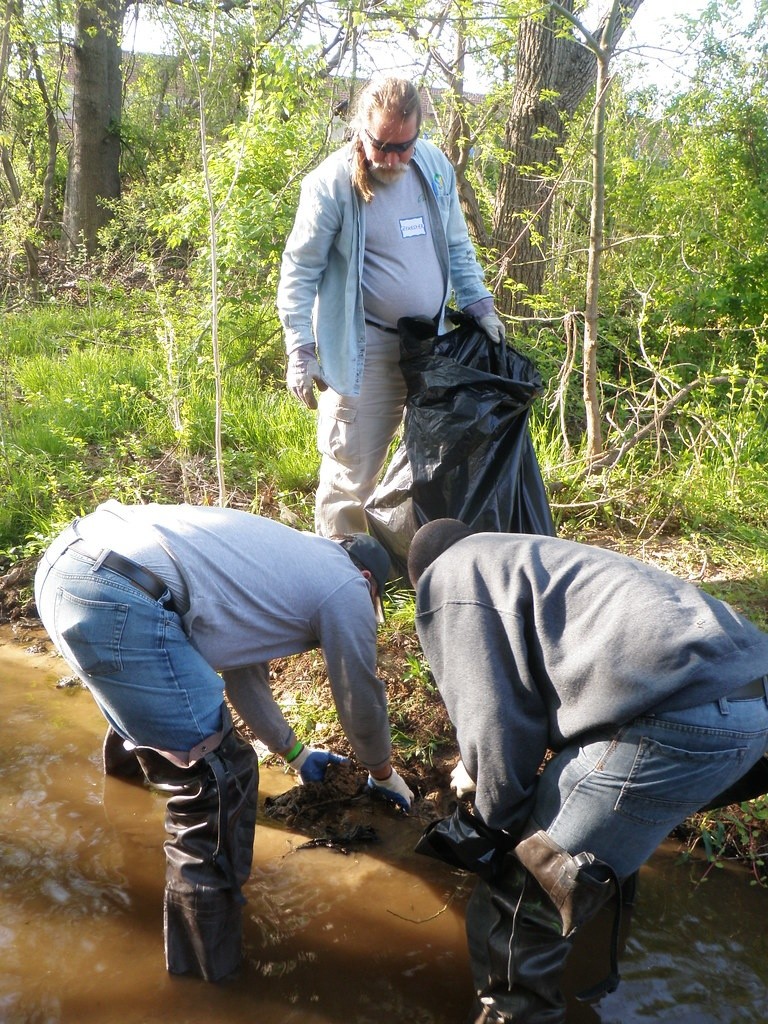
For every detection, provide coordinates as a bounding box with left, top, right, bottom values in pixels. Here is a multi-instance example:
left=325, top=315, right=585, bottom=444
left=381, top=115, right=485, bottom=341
left=726, top=678, right=765, bottom=700
left=64, top=520, right=177, bottom=612
left=365, top=314, right=441, bottom=333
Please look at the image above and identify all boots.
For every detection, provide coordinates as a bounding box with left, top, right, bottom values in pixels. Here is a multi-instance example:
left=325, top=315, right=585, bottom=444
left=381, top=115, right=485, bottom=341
left=103, top=698, right=258, bottom=981
left=415, top=799, right=640, bottom=1024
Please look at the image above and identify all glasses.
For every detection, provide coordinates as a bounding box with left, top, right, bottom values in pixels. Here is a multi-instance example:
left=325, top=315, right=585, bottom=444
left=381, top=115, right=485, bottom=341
left=365, top=129, right=419, bottom=153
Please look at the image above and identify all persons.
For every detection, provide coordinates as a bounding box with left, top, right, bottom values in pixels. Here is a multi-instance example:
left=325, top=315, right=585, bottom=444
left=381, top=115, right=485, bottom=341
left=406, top=519, right=768, bottom=1024
left=34, top=497, right=415, bottom=983
left=275, top=76, right=506, bottom=547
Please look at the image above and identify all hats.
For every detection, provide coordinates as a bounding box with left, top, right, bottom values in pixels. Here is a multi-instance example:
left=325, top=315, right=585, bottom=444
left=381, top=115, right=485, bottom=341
left=340, top=533, right=391, bottom=622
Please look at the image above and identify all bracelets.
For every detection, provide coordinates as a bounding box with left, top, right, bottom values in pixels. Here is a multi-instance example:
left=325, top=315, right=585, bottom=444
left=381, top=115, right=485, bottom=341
left=284, top=742, right=303, bottom=762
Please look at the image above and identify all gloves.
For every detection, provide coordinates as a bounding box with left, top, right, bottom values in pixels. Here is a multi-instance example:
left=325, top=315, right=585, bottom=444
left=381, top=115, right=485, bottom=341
left=367, top=765, right=415, bottom=813
left=450, top=762, right=476, bottom=799
left=286, top=343, right=328, bottom=409
left=287, top=744, right=352, bottom=786
left=462, top=298, right=505, bottom=344
